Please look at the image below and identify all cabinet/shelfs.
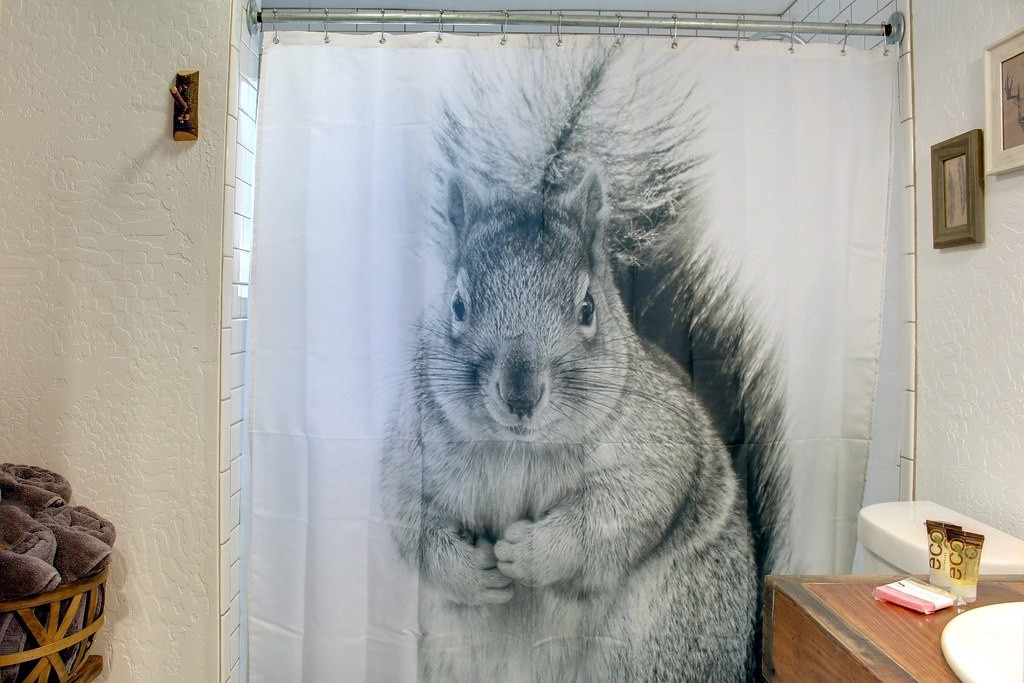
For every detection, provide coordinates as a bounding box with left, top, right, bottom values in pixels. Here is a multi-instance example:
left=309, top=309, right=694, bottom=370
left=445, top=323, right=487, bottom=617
left=761, top=577, right=882, bottom=683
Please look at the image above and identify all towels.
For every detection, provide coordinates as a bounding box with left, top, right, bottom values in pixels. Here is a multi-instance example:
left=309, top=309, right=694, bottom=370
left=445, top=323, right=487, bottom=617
left=0, top=460, right=116, bottom=683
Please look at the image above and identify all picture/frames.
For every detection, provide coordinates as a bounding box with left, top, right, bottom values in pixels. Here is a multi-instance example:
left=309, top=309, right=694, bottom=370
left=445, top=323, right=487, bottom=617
left=930, top=128, right=985, bottom=249
left=983, top=26, right=1024, bottom=175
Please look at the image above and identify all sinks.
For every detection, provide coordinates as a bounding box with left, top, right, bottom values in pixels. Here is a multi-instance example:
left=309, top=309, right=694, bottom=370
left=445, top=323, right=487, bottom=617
left=940, top=599, right=1024, bottom=683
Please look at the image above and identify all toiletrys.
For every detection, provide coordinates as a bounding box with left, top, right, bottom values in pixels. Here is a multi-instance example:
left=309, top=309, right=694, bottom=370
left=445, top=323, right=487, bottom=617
left=923, top=516, right=987, bottom=604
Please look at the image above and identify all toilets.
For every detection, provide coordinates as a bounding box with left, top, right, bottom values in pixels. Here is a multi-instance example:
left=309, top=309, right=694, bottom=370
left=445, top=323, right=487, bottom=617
left=849, top=500, right=1024, bottom=579
